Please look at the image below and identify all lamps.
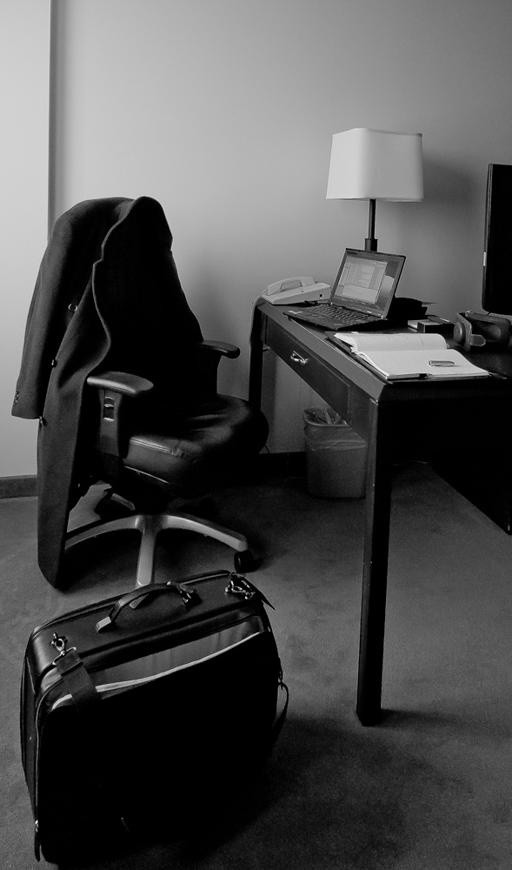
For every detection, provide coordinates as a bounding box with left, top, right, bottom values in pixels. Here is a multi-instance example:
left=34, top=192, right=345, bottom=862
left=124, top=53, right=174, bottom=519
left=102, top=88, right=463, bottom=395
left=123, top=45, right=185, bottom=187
left=325, top=126, right=425, bottom=252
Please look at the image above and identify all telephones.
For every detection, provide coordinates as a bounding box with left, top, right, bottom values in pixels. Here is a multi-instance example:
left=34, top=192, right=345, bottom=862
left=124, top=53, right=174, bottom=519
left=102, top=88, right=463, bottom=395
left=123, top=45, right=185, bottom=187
left=262, top=276, right=331, bottom=305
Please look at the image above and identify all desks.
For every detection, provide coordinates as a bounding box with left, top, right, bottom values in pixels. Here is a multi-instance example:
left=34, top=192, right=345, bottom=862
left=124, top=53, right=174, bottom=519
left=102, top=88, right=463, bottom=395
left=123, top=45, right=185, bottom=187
left=249, top=288, right=511, bottom=725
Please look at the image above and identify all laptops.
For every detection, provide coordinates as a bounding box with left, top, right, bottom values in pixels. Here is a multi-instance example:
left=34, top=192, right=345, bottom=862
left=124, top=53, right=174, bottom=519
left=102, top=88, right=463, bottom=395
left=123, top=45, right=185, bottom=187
left=283, top=248, right=406, bottom=331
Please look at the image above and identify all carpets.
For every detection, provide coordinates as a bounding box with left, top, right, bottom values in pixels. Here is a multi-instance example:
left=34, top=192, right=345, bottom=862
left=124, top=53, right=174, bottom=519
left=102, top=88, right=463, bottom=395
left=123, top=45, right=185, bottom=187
left=0, top=464, right=510, bottom=870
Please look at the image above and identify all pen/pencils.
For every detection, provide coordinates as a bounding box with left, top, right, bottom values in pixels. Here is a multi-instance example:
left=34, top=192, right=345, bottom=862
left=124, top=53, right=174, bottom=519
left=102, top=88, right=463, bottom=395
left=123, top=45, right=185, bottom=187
left=429, top=361, right=455, bottom=366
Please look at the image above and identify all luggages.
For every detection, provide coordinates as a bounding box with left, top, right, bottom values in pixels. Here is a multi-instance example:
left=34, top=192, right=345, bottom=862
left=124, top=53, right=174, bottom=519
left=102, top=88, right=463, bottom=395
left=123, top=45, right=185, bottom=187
left=18, top=571, right=291, bottom=864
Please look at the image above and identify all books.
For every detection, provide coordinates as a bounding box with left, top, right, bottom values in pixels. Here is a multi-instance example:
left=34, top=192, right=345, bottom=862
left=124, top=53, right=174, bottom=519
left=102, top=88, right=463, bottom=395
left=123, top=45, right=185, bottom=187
left=325, top=331, right=491, bottom=385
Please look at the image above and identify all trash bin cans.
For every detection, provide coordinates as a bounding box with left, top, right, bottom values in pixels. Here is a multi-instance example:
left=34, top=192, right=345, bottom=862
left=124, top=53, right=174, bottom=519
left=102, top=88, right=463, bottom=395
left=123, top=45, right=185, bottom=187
left=303, top=407, right=369, bottom=498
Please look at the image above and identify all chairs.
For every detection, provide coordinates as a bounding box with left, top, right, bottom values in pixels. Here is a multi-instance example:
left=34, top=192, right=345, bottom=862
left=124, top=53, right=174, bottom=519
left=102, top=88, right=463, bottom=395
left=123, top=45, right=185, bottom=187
left=44, top=196, right=269, bottom=598
